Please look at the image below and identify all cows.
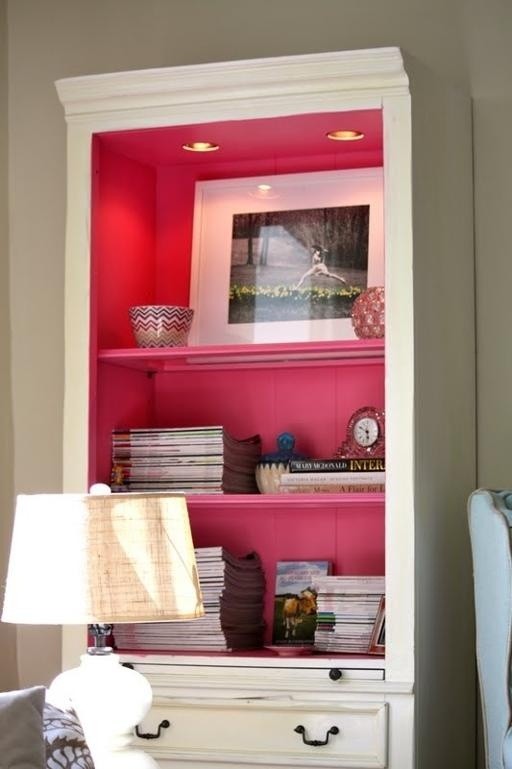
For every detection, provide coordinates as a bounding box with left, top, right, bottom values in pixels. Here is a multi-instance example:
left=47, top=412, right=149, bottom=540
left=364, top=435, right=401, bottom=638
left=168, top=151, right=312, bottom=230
left=280, top=585, right=316, bottom=639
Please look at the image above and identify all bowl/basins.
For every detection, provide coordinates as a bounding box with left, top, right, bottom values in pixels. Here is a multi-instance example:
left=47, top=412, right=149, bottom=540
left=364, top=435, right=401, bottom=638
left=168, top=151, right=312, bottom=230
left=128, top=305, right=194, bottom=348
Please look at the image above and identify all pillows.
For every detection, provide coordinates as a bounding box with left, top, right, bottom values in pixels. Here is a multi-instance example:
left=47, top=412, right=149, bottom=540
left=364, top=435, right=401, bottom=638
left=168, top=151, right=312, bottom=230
left=43, top=701, right=96, bottom=769
left=0, top=684, right=47, bottom=769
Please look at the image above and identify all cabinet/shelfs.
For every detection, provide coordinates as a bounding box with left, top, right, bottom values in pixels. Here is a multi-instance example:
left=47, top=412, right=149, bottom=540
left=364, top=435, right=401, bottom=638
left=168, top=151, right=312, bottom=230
left=56, top=46, right=483, bottom=769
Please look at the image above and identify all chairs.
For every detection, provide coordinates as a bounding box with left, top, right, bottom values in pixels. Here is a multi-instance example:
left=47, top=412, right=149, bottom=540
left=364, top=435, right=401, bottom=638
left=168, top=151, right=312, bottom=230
left=462, top=485, right=512, bottom=769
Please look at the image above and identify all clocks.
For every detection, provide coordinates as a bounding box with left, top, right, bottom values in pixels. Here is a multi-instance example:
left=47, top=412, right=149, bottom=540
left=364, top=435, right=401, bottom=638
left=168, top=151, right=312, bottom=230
left=333, top=401, right=385, bottom=461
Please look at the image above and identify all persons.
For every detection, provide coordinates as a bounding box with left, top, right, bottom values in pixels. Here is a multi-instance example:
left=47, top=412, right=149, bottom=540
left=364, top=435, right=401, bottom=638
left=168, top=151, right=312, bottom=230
left=297, top=246, right=346, bottom=292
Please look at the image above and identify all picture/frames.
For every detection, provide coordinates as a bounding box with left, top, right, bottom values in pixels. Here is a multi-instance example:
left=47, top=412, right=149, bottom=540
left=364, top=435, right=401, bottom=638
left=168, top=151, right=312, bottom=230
left=182, top=166, right=383, bottom=346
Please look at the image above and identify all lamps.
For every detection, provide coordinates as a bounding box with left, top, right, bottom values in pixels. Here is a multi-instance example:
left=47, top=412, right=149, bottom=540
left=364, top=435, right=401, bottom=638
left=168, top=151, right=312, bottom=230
left=0, top=481, right=209, bottom=769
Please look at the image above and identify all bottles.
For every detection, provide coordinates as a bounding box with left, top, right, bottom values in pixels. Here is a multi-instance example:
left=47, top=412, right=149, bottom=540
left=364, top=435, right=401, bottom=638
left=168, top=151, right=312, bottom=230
left=254, top=431, right=303, bottom=495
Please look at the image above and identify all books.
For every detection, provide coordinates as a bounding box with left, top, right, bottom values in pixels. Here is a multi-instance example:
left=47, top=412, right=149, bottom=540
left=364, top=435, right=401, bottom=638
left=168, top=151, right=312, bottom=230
left=109, top=425, right=261, bottom=495
left=113, top=548, right=384, bottom=653
